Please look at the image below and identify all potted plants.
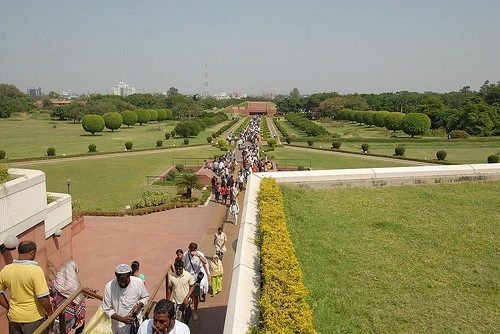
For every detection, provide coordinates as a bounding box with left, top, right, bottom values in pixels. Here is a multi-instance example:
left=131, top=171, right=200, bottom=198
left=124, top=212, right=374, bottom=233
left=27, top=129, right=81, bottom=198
left=72, top=199, right=85, bottom=236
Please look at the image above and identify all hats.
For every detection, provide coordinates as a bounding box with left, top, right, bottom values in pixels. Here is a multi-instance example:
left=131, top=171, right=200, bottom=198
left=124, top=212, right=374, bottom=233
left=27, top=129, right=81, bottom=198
left=115, top=264, right=131, bottom=274
left=213, top=255, right=218, bottom=258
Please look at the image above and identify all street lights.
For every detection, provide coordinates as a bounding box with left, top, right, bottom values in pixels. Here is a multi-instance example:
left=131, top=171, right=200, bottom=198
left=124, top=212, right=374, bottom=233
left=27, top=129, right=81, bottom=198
left=67, top=178, right=70, bottom=194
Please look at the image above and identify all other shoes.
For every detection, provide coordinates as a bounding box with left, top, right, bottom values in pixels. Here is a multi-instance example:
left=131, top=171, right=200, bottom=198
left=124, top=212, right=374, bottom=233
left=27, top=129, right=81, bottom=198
left=193, top=314, right=198, bottom=321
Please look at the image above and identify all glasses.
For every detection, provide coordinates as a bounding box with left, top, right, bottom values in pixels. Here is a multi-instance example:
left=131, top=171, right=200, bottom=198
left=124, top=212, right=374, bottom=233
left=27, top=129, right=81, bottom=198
left=175, top=268, right=182, bottom=270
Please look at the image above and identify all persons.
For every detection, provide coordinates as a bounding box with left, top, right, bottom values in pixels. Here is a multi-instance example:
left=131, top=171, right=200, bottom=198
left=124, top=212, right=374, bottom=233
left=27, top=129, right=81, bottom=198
left=229, top=200, right=239, bottom=225
left=0, top=240, right=210, bottom=334
left=213, top=227, right=227, bottom=261
left=180, top=187, right=191, bottom=199
left=209, top=255, right=223, bottom=296
left=204, top=114, right=271, bottom=206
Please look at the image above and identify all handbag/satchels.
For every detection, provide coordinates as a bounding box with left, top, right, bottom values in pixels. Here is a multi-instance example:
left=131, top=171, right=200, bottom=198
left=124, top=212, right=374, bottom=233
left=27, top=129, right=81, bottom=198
left=197, top=271, right=204, bottom=280
